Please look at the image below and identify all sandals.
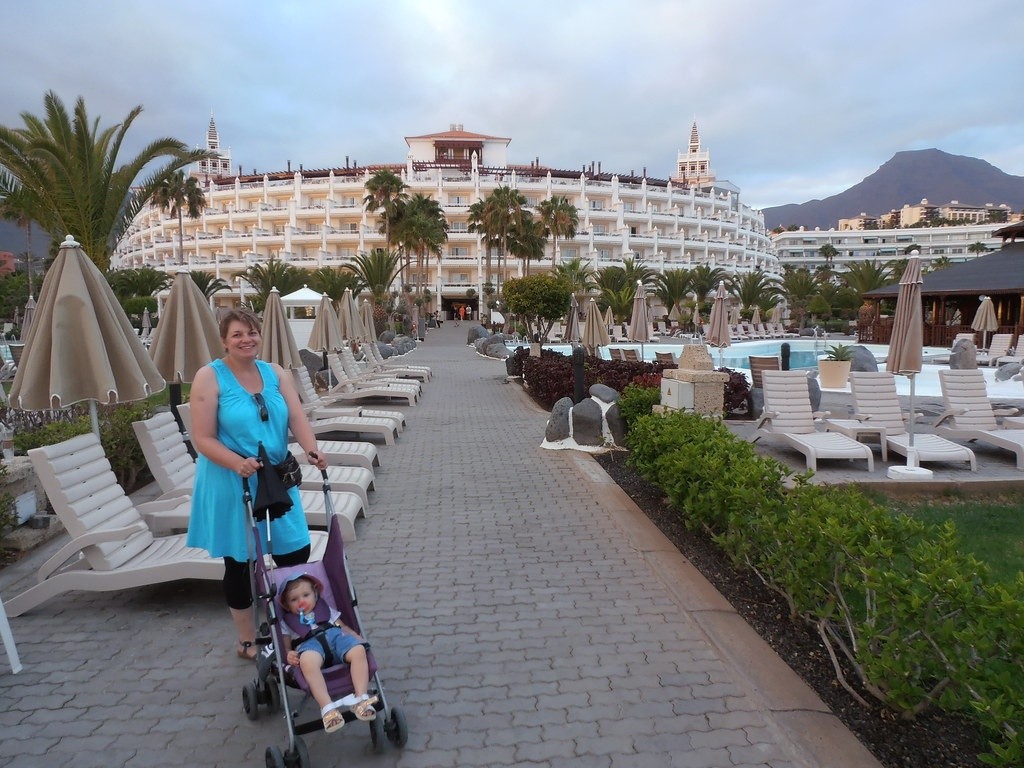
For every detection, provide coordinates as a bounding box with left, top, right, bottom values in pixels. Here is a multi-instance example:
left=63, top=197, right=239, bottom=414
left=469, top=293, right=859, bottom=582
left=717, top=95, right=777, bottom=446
left=350, top=695, right=377, bottom=721
left=322, top=703, right=345, bottom=733
left=237, top=642, right=258, bottom=658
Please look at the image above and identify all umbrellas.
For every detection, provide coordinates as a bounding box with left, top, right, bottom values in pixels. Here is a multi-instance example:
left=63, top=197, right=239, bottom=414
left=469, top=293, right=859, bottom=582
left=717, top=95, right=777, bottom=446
left=6, top=235, right=168, bottom=443
left=751, top=305, right=761, bottom=330
left=563, top=292, right=582, bottom=350
left=337, top=286, right=369, bottom=341
left=307, top=291, right=345, bottom=353
left=772, top=304, right=781, bottom=322
left=691, top=302, right=701, bottom=332
left=704, top=280, right=731, bottom=370
left=145, top=269, right=228, bottom=384
left=971, top=296, right=1000, bottom=350
left=730, top=306, right=739, bottom=325
left=20, top=294, right=36, bottom=345
left=13, top=306, right=19, bottom=329
left=360, top=298, right=377, bottom=344
left=628, top=284, right=650, bottom=362
left=257, top=286, right=304, bottom=370
left=603, top=305, right=616, bottom=335
left=142, top=306, right=152, bottom=328
left=885, top=249, right=926, bottom=447
left=583, top=296, right=611, bottom=357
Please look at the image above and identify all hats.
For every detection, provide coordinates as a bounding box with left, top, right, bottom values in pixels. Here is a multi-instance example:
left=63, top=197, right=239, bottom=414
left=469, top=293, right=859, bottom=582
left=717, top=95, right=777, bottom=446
left=279, top=572, right=322, bottom=608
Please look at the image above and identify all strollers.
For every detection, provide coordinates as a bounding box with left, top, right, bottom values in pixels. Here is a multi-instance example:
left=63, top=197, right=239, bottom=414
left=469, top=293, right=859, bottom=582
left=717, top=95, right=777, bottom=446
left=241, top=452, right=409, bottom=768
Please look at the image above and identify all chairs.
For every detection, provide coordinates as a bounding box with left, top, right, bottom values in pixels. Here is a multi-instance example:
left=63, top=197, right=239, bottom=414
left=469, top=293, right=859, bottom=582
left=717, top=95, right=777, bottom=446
left=542, top=322, right=678, bottom=343
left=2, top=343, right=432, bottom=618
left=655, top=352, right=673, bottom=363
left=850, top=371, right=977, bottom=472
left=749, top=356, right=779, bottom=391
left=609, top=348, right=622, bottom=360
left=702, top=323, right=800, bottom=340
left=746, top=370, right=874, bottom=473
left=932, top=369, right=1024, bottom=471
left=622, top=349, right=638, bottom=361
left=952, top=333, right=1024, bottom=368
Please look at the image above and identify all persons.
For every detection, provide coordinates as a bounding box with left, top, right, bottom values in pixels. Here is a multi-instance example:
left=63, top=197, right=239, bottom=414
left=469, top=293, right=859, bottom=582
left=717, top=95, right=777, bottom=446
left=185, top=309, right=329, bottom=659
left=459, top=305, right=465, bottom=321
left=466, top=305, right=472, bottom=321
left=454, top=307, right=459, bottom=327
left=274, top=571, right=379, bottom=733
left=435, top=310, right=441, bottom=328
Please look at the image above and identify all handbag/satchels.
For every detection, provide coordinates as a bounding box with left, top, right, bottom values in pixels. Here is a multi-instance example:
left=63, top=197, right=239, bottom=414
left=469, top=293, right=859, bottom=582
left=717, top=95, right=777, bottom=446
left=275, top=449, right=301, bottom=490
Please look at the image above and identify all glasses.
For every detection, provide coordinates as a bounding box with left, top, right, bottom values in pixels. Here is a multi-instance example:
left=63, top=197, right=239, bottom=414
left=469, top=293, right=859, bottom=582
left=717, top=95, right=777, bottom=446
left=252, top=393, right=269, bottom=422
